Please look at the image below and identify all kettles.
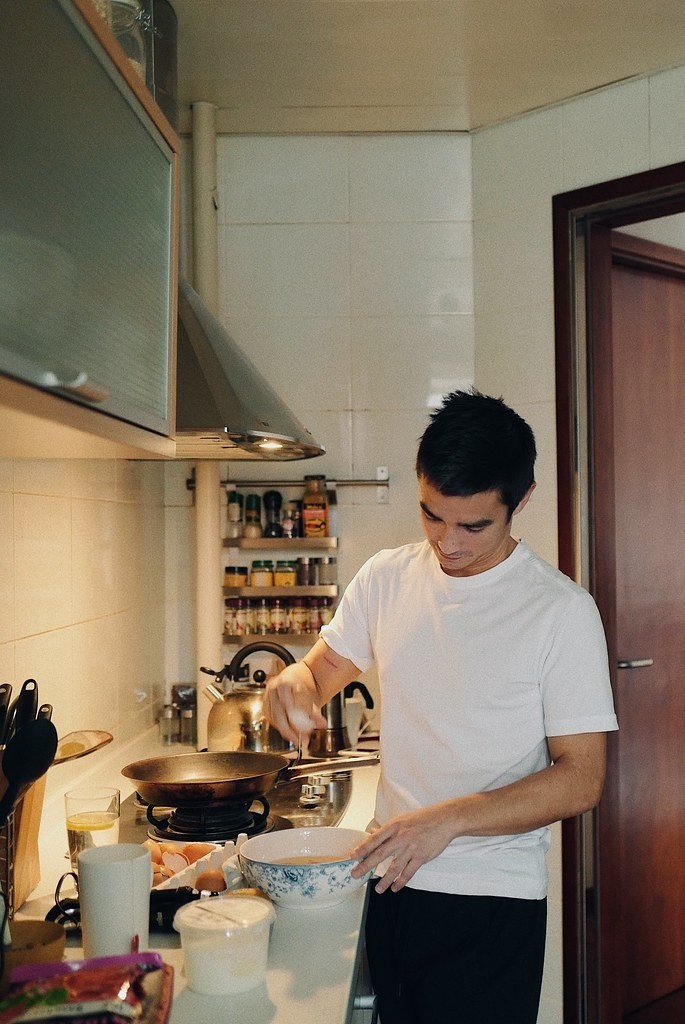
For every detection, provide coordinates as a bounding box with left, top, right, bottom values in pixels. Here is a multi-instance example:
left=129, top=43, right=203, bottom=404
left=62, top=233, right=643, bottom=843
left=203, top=641, right=296, bottom=755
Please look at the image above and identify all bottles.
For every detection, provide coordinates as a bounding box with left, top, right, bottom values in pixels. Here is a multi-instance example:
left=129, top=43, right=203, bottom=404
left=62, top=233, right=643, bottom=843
left=292, top=511, right=303, bottom=538
left=180, top=709, right=197, bottom=746
left=224, top=555, right=337, bottom=635
left=159, top=707, right=181, bottom=745
left=302, top=475, right=329, bottom=538
left=282, top=510, right=293, bottom=538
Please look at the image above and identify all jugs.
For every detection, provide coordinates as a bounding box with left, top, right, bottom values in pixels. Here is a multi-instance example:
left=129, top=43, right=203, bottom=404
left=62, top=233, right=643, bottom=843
left=308, top=682, right=374, bottom=756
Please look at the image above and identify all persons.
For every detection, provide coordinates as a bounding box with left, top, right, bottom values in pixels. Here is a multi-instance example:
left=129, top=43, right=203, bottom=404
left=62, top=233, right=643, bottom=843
left=264, top=388, right=619, bottom=1024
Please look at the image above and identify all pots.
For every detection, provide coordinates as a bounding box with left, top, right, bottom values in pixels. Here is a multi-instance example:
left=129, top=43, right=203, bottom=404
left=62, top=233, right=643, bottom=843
left=121, top=751, right=380, bottom=805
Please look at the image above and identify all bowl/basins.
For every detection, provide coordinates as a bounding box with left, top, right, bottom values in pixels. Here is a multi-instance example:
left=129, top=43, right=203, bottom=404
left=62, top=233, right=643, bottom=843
left=241, top=827, right=378, bottom=907
left=173, top=895, right=277, bottom=995
left=4, top=921, right=65, bottom=969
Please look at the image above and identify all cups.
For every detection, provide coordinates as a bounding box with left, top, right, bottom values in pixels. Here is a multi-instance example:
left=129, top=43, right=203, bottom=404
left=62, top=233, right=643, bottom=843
left=66, top=788, right=119, bottom=875
left=345, top=699, right=376, bottom=746
left=76, top=844, right=154, bottom=959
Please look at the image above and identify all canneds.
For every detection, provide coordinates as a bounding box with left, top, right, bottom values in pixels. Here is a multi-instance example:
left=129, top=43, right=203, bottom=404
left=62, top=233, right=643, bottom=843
left=225, top=595, right=333, bottom=637
left=225, top=556, right=337, bottom=588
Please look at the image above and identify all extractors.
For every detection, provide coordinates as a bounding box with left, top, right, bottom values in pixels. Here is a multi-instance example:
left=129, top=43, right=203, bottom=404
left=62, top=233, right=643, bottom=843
left=127, top=273, right=325, bottom=458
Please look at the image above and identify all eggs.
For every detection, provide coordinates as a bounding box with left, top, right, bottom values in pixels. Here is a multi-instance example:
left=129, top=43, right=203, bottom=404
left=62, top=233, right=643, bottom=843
left=195, top=869, right=226, bottom=893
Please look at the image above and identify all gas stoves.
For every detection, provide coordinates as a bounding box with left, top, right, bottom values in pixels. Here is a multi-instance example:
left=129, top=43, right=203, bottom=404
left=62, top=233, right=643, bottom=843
left=119, top=748, right=355, bottom=846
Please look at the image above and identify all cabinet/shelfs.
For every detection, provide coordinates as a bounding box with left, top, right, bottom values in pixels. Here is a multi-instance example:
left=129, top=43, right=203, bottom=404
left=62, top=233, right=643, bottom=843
left=0, top=0, right=181, bottom=461
left=221, top=536, right=339, bottom=648
left=349, top=944, right=381, bottom=1024
